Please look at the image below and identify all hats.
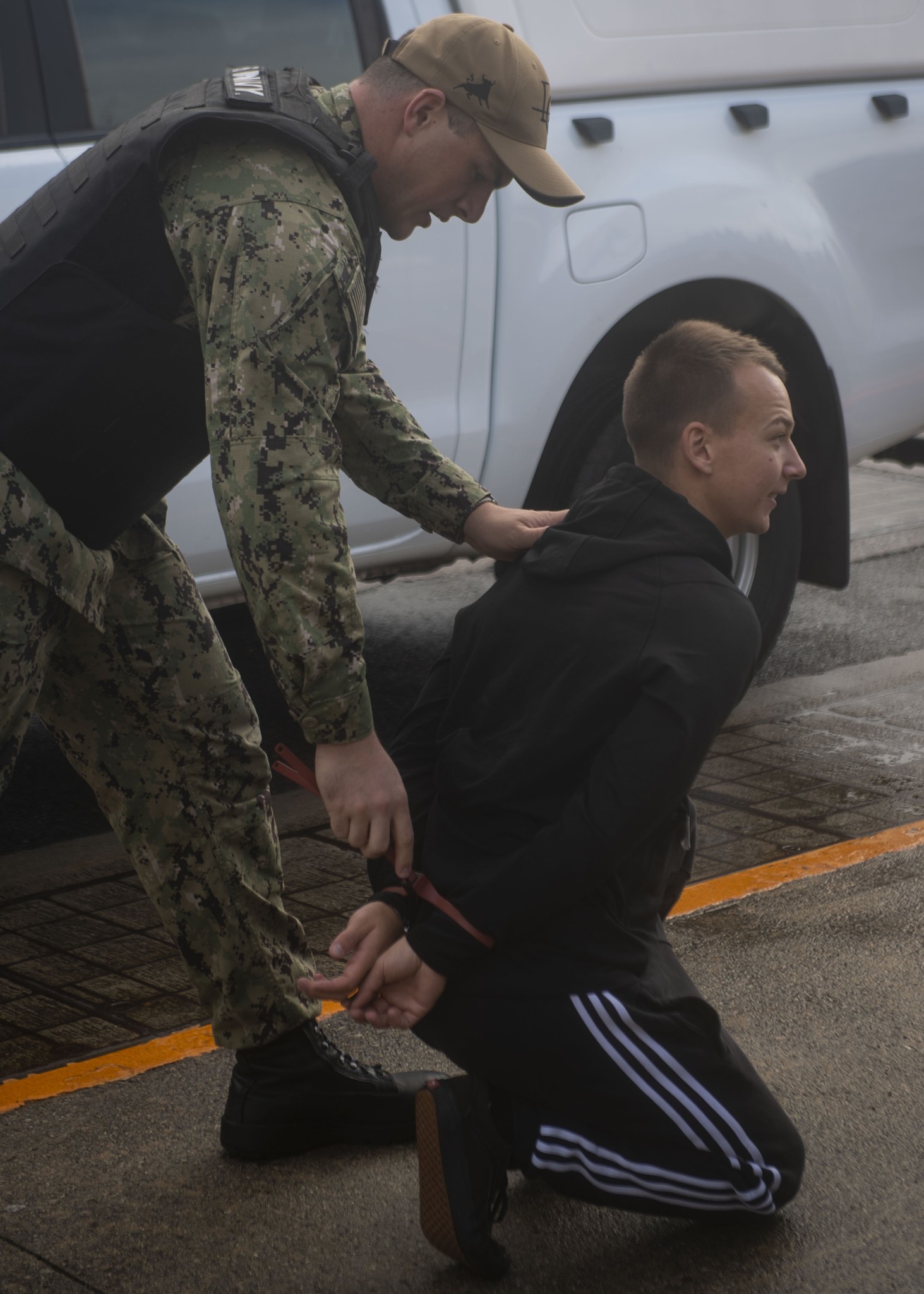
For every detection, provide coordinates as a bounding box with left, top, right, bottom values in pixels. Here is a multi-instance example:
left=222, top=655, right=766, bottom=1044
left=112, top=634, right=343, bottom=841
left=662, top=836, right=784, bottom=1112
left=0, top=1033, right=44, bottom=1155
left=382, top=14, right=585, bottom=208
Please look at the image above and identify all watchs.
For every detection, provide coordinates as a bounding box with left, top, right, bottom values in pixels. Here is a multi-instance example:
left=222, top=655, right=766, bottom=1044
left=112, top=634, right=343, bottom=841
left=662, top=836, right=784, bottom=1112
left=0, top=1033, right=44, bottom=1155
left=461, top=494, right=498, bottom=544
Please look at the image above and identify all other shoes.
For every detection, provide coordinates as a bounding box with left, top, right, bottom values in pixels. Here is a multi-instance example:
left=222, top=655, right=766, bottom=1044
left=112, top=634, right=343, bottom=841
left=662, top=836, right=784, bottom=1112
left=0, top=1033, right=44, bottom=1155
left=412, top=1071, right=494, bottom=1258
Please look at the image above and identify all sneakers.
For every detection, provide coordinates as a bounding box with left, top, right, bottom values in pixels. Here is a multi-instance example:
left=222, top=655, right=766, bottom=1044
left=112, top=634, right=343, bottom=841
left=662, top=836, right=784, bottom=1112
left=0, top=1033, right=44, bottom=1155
left=219, top=1016, right=453, bottom=1156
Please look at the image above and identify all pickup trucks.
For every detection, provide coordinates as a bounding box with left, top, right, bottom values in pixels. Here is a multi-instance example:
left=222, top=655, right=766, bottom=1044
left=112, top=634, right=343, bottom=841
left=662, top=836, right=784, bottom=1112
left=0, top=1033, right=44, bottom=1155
left=0, top=1, right=923, bottom=684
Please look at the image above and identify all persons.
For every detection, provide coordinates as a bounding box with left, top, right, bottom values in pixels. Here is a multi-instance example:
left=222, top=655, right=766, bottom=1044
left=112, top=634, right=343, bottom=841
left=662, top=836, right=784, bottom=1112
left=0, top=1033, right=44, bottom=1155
left=0, top=14, right=586, bottom=1161
left=299, top=319, right=806, bottom=1280
